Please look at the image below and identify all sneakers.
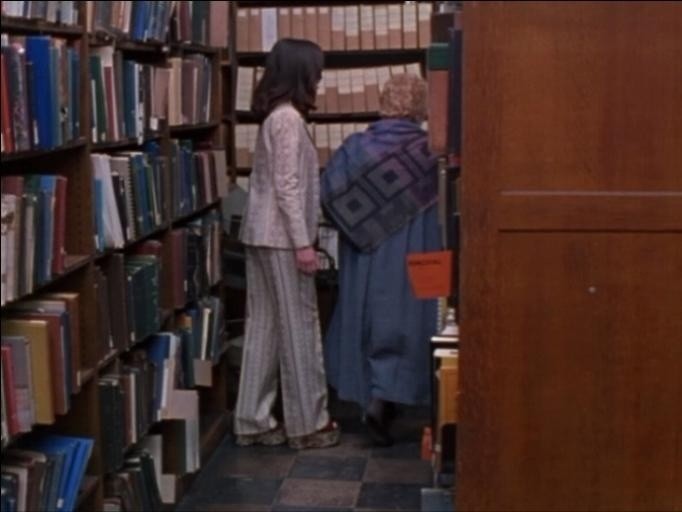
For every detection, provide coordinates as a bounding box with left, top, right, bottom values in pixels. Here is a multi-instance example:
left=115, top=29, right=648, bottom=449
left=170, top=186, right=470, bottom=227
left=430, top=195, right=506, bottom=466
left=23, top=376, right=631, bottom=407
left=364, top=401, right=395, bottom=449
left=234, top=415, right=289, bottom=447
left=285, top=417, right=343, bottom=450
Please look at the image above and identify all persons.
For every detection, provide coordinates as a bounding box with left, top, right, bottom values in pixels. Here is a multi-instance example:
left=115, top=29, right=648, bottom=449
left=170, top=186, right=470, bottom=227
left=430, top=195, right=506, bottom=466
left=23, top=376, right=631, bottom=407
left=315, top=68, right=450, bottom=458
left=233, top=36, right=340, bottom=452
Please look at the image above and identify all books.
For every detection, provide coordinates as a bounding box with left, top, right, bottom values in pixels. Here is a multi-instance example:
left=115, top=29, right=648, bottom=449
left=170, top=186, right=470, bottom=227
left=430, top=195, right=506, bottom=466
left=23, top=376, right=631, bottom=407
left=313, top=207, right=342, bottom=270
left=404, top=7, right=456, bottom=512
left=258, top=3, right=433, bottom=170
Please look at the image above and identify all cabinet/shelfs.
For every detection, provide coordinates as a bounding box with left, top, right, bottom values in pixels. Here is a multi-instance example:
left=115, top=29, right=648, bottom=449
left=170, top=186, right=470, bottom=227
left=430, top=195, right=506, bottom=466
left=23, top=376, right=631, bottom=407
left=454, top=1, right=681, bottom=510
left=233, top=3, right=433, bottom=283
left=1, top=0, right=231, bottom=510
